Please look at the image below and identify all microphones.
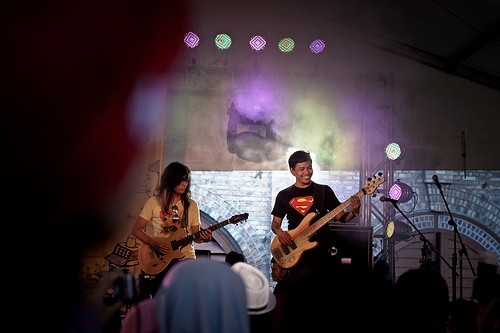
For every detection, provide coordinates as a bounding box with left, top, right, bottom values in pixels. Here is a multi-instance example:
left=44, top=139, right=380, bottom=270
left=432, top=175, right=441, bottom=190
left=379, top=196, right=399, bottom=203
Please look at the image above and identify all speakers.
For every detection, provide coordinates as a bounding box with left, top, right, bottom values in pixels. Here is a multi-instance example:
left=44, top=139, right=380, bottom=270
left=327, top=226, right=373, bottom=279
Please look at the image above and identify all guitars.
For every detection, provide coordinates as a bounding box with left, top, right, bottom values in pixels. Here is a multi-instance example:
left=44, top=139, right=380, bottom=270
left=138, top=213, right=249, bottom=275
left=270, top=172, right=385, bottom=268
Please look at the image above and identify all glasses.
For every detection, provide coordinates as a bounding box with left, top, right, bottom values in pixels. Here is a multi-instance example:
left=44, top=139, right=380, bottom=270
left=172, top=205, right=179, bottom=223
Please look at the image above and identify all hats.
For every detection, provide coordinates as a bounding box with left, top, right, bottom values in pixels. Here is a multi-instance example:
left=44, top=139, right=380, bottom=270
left=230, top=262, right=276, bottom=315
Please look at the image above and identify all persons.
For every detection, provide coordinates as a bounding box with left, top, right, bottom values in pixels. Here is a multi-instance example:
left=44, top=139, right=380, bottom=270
left=132, top=161, right=213, bottom=262
left=271, top=150, right=361, bottom=278
left=119, top=251, right=500, bottom=333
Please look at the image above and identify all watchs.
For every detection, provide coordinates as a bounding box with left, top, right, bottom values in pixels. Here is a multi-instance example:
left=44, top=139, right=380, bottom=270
left=351, top=210, right=360, bottom=217
